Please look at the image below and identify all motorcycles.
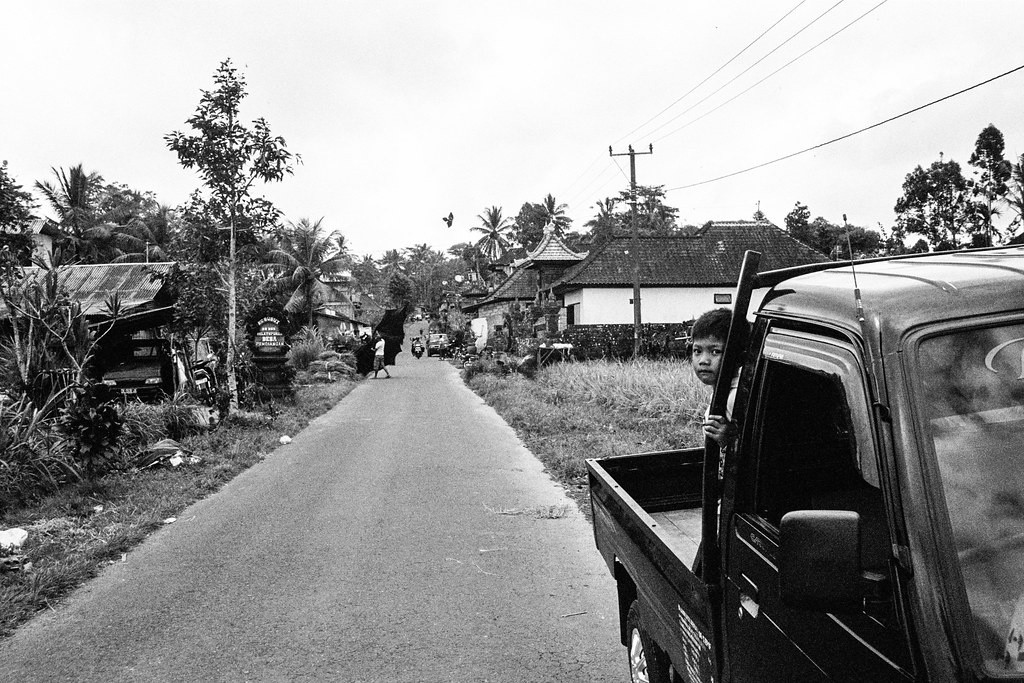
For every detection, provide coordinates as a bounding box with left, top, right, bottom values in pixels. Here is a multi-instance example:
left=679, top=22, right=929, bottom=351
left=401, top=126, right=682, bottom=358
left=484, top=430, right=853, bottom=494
left=187, top=359, right=220, bottom=403
left=413, top=343, right=423, bottom=359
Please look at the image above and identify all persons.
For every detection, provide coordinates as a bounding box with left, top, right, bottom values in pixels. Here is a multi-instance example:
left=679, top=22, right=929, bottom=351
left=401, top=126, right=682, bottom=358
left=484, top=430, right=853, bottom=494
left=371, top=330, right=391, bottom=379
left=438, top=333, right=460, bottom=360
left=344, top=327, right=368, bottom=344
left=692, top=308, right=751, bottom=542
left=413, top=337, right=423, bottom=348
left=419, top=328, right=423, bottom=336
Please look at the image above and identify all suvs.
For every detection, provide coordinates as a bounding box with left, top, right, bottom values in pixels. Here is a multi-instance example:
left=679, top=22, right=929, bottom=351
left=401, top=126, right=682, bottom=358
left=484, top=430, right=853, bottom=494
left=426, top=334, right=451, bottom=357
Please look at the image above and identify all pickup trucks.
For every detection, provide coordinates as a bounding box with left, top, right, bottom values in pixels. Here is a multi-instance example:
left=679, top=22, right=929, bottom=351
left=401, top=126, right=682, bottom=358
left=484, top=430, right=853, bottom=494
left=585, top=212, right=1024, bottom=683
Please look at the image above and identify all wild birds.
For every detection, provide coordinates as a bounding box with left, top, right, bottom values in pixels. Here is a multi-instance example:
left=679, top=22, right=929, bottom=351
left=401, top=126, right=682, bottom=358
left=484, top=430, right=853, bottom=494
left=443, top=212, right=453, bottom=227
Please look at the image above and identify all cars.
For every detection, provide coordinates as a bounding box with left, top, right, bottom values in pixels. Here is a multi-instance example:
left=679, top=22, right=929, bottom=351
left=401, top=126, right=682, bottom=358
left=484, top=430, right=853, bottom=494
left=425, top=315, right=430, bottom=319
left=415, top=314, right=423, bottom=321
left=101, top=339, right=186, bottom=403
